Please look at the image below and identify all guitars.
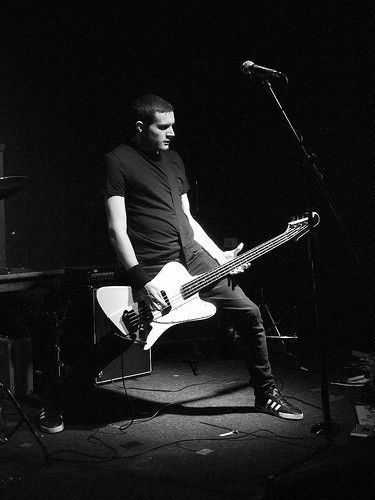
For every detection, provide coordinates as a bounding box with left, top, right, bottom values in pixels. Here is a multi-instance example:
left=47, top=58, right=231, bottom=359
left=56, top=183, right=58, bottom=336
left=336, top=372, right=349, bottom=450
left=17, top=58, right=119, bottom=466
left=96, top=211, right=321, bottom=351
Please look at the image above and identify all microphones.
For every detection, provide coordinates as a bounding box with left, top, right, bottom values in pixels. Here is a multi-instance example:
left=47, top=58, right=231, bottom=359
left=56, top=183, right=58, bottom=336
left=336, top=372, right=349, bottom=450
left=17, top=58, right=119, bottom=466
left=243, top=61, right=281, bottom=77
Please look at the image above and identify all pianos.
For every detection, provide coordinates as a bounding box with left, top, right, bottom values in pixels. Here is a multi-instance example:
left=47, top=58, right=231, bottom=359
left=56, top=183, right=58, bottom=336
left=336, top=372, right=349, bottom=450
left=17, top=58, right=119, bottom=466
left=66, top=266, right=117, bottom=293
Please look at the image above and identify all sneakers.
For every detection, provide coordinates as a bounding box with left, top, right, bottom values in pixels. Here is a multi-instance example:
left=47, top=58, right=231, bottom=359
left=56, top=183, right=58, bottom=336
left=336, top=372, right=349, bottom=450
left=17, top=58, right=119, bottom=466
left=38, top=398, right=64, bottom=433
left=254, top=382, right=303, bottom=420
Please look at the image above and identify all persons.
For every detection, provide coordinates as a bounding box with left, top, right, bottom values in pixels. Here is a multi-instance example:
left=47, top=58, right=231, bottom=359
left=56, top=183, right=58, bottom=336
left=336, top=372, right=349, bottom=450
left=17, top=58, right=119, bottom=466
left=28, top=95, right=305, bottom=434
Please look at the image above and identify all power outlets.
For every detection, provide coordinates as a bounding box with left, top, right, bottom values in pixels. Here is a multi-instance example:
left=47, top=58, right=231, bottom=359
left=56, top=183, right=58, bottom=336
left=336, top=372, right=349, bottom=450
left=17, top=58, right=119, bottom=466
left=224, top=238, right=238, bottom=247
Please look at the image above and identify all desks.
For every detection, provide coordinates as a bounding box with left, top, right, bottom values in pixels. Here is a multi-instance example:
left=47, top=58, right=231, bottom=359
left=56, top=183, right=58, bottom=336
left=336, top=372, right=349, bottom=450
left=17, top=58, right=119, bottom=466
left=0, top=268, right=67, bottom=396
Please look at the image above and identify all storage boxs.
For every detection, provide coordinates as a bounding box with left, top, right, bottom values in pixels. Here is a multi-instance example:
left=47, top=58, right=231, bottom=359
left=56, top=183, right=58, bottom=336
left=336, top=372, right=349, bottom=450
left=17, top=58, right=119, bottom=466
left=77, top=284, right=152, bottom=384
left=0, top=337, right=33, bottom=398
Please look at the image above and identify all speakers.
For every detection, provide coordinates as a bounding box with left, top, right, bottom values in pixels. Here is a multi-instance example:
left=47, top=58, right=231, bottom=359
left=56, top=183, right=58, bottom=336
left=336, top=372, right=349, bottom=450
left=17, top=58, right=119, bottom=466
left=62, top=266, right=152, bottom=385
